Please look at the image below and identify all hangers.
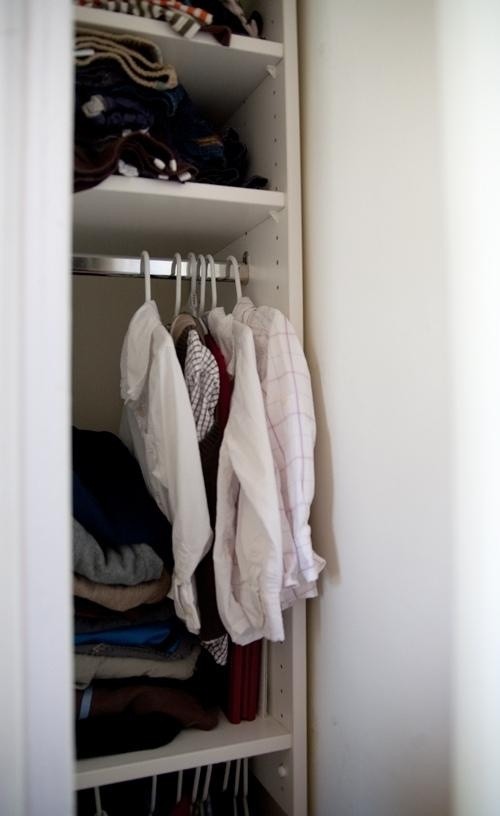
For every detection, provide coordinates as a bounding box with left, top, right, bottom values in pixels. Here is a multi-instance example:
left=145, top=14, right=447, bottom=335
left=92, top=758, right=253, bottom=815
left=137, top=249, right=244, bottom=316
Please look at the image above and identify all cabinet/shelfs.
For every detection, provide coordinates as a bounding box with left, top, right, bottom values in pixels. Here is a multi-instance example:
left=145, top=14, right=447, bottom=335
left=75, top=1, right=310, bottom=816
